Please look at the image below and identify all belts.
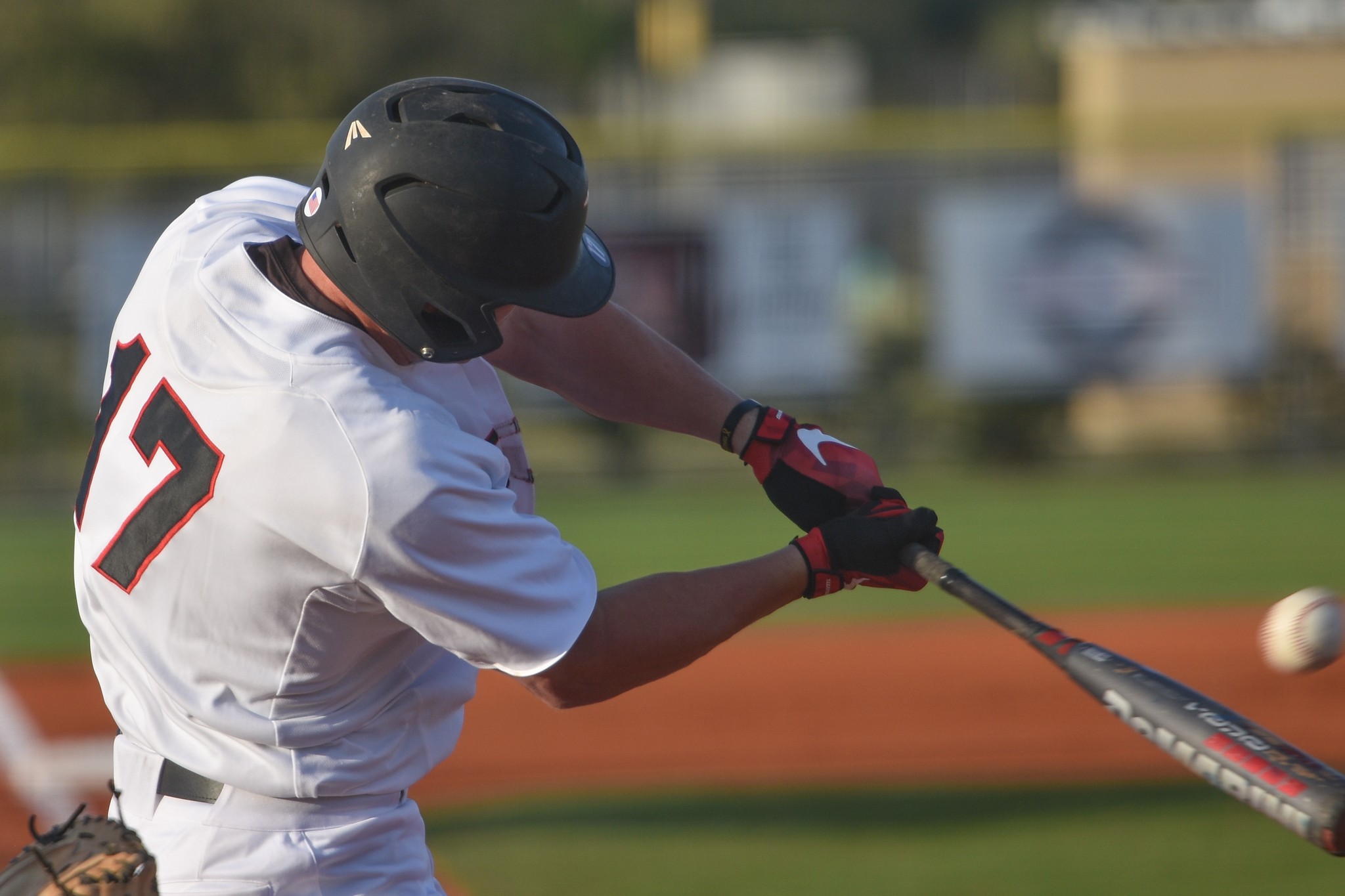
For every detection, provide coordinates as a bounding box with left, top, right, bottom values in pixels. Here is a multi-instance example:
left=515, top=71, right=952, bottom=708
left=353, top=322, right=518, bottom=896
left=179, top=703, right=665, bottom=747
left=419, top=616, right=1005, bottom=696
left=155, top=760, right=220, bottom=804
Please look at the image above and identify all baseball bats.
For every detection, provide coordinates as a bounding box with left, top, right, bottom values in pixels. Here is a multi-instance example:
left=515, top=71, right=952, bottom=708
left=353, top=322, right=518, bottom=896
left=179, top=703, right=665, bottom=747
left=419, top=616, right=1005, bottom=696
left=900, top=543, right=1344, bottom=858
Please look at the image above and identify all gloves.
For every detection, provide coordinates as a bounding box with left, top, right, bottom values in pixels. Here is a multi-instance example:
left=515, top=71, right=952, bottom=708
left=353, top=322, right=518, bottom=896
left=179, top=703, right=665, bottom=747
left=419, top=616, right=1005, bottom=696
left=790, top=482, right=947, bottom=597
left=737, top=404, right=888, bottom=536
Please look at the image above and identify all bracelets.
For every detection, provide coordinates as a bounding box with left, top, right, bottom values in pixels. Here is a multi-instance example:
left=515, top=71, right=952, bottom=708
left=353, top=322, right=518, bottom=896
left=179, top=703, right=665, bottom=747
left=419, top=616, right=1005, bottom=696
left=720, top=398, right=762, bottom=453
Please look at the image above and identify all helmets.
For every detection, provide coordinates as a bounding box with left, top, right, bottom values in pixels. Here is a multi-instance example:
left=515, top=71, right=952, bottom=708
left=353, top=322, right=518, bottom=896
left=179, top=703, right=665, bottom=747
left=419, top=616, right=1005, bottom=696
left=295, top=72, right=617, bottom=360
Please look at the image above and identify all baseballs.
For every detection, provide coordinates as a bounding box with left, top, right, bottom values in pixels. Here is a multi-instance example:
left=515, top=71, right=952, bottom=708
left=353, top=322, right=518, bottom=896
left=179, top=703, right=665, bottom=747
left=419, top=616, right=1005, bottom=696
left=1257, top=587, right=1344, bottom=675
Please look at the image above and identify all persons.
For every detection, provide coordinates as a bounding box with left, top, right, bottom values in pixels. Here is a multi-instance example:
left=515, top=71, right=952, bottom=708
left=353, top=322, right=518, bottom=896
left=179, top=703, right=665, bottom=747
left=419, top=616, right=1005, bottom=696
left=75, top=74, right=944, bottom=896
